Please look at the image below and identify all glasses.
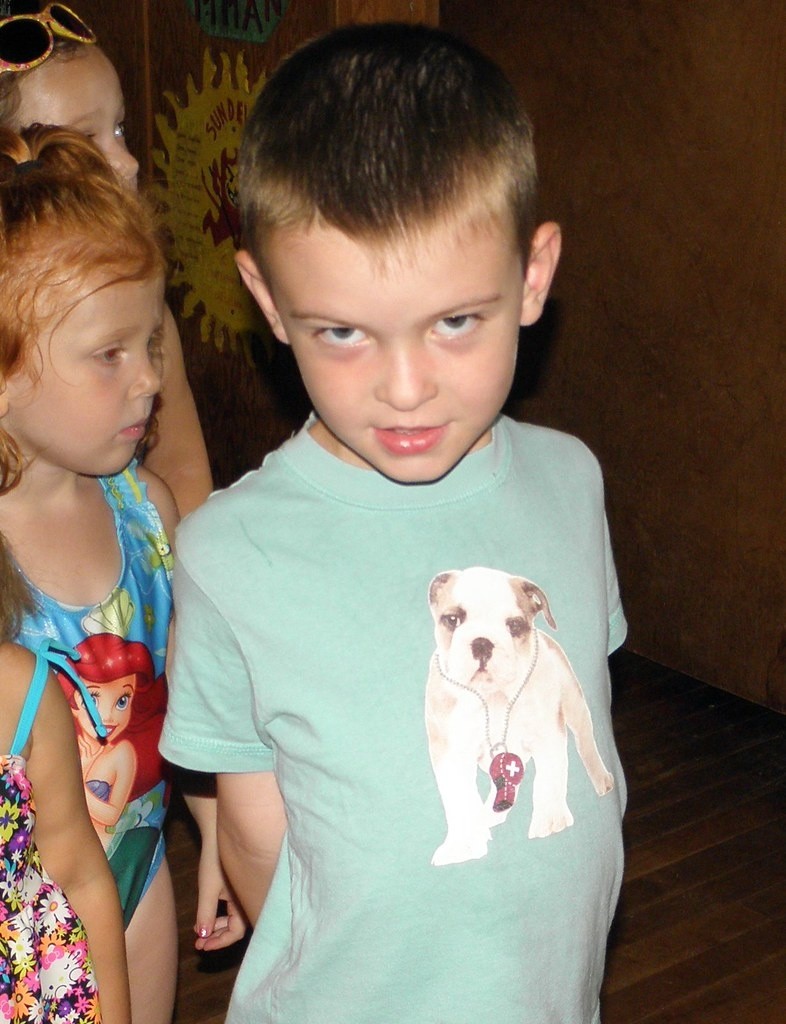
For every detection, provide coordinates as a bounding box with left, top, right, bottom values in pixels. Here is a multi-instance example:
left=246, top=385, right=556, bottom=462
left=0, top=3, right=97, bottom=74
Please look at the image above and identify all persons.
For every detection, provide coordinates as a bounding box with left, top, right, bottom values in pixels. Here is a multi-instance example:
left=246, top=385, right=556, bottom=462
left=0, top=0, right=246, bottom=1024
left=160, top=23, right=629, bottom=1024
